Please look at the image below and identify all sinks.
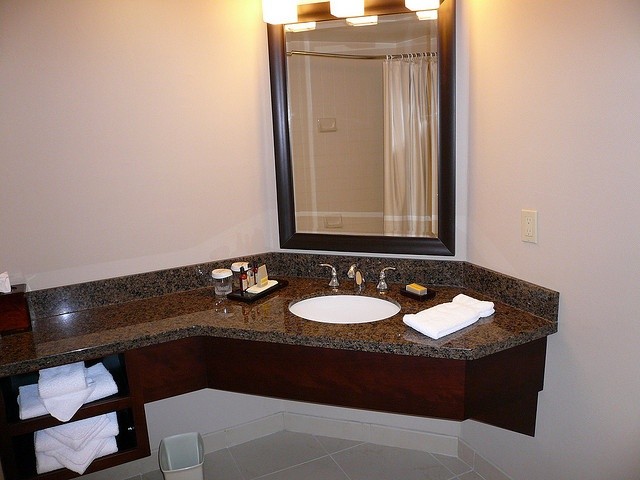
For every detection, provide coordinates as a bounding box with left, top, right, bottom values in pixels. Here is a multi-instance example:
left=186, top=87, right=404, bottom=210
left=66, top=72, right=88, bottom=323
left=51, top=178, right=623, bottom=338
left=288, top=293, right=401, bottom=325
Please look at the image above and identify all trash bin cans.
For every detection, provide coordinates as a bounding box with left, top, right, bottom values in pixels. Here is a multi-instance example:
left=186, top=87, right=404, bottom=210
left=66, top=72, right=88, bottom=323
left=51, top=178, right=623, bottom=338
left=159, top=432, right=205, bottom=479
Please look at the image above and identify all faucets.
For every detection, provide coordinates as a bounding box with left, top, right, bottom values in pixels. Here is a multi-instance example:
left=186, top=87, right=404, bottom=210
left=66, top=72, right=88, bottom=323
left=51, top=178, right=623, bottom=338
left=320, top=263, right=395, bottom=295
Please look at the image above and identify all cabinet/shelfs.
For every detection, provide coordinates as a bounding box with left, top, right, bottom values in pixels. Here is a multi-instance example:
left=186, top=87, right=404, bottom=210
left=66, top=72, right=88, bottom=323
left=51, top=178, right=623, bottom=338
left=2, top=353, right=152, bottom=480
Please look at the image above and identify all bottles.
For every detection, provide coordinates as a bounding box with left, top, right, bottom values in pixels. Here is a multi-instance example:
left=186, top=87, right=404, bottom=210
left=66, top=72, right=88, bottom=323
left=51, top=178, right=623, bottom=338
left=247, top=264, right=255, bottom=287
left=230, top=262, right=248, bottom=290
left=254, top=262, right=259, bottom=283
left=240, top=267, right=247, bottom=292
left=212, top=268, right=233, bottom=296
